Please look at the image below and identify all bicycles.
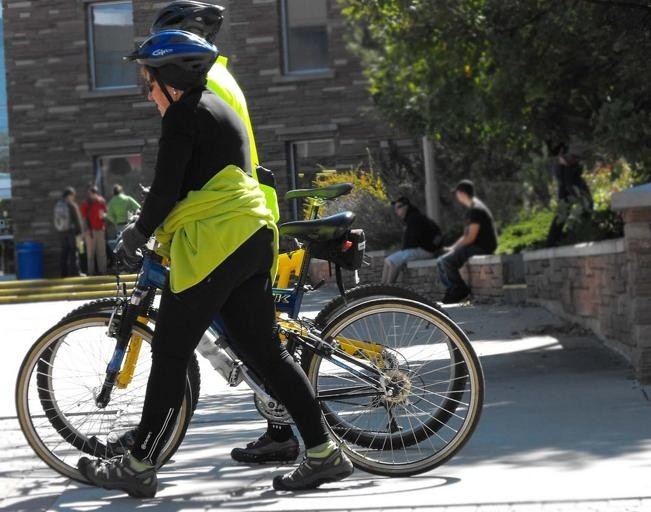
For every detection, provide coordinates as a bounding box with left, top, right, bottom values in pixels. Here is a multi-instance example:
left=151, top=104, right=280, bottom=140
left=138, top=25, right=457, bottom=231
left=15, top=209, right=483, bottom=487
left=35, top=183, right=467, bottom=461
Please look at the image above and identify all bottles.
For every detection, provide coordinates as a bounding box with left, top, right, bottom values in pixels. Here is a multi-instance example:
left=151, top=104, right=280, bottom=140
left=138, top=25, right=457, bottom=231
left=391, top=196, right=410, bottom=206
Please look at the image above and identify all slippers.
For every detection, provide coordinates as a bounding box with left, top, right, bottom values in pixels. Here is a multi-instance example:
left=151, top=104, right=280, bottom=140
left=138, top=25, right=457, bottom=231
left=422, top=213, right=443, bottom=251
left=53, top=200, right=72, bottom=233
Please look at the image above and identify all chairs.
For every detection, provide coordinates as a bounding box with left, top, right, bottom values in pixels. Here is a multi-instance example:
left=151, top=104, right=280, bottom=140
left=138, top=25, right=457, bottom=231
left=272, top=440, right=355, bottom=492
left=76, top=448, right=159, bottom=500
left=441, top=288, right=467, bottom=304
left=230, top=431, right=300, bottom=464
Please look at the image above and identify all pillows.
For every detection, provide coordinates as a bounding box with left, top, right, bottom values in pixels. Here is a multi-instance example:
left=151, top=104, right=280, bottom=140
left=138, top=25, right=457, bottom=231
left=148, top=0, right=227, bottom=34
left=119, top=26, right=222, bottom=73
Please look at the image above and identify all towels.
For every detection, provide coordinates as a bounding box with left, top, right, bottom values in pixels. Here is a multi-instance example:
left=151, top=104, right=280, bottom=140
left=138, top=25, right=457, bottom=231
left=15, top=240, right=45, bottom=279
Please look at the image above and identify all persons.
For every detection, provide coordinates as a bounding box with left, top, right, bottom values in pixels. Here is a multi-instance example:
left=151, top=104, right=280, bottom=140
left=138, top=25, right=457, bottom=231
left=77, top=32, right=353, bottom=500
left=49, top=186, right=86, bottom=279
left=378, top=196, right=444, bottom=284
left=105, top=185, right=142, bottom=234
left=435, top=179, right=498, bottom=303
left=77, top=185, right=110, bottom=276
left=83, top=1, right=302, bottom=463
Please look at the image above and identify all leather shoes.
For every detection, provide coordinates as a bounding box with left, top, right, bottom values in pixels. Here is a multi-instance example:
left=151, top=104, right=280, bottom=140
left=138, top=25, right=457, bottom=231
left=120, top=224, right=148, bottom=254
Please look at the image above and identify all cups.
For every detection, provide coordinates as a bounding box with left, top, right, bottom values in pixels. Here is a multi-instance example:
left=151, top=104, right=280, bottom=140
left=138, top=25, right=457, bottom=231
left=144, top=77, right=156, bottom=92
